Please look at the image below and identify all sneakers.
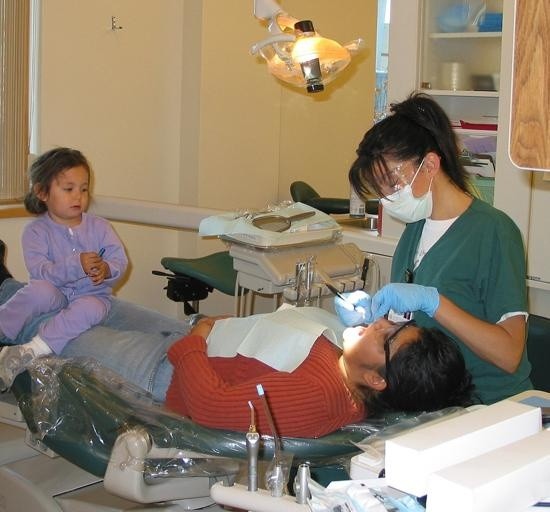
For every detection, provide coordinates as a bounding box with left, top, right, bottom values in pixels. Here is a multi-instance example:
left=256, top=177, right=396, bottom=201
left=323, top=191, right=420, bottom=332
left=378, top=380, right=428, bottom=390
left=0, top=344, right=36, bottom=393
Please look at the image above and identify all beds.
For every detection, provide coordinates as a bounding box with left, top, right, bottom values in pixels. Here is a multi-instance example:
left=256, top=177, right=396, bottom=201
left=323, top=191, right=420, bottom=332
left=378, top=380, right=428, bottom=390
left=0, top=351, right=468, bottom=503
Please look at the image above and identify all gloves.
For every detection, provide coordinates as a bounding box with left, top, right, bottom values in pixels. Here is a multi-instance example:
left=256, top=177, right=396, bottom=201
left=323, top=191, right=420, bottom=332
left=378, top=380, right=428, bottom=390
left=371, top=283, right=440, bottom=322
left=334, top=289, right=372, bottom=328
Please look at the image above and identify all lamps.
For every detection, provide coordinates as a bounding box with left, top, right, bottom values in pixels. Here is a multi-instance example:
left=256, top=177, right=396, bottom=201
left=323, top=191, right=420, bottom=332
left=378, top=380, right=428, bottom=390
left=249, top=1, right=351, bottom=94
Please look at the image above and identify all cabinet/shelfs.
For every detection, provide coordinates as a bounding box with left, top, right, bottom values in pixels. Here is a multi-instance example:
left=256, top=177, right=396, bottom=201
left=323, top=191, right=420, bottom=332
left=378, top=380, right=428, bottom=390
left=380, top=0, right=533, bottom=290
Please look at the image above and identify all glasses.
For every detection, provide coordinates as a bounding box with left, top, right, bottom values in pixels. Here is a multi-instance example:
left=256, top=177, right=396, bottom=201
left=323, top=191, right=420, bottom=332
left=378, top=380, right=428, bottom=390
left=375, top=318, right=418, bottom=388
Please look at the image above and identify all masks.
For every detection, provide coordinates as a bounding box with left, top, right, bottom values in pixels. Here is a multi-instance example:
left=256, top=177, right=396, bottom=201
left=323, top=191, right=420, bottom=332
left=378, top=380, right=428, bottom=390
left=378, top=186, right=434, bottom=224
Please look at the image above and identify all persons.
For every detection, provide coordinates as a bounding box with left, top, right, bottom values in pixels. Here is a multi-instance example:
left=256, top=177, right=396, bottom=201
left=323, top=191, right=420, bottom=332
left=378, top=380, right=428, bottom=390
left=0, top=241, right=483, bottom=440
left=0, top=144, right=129, bottom=394
left=332, top=86, right=536, bottom=409
